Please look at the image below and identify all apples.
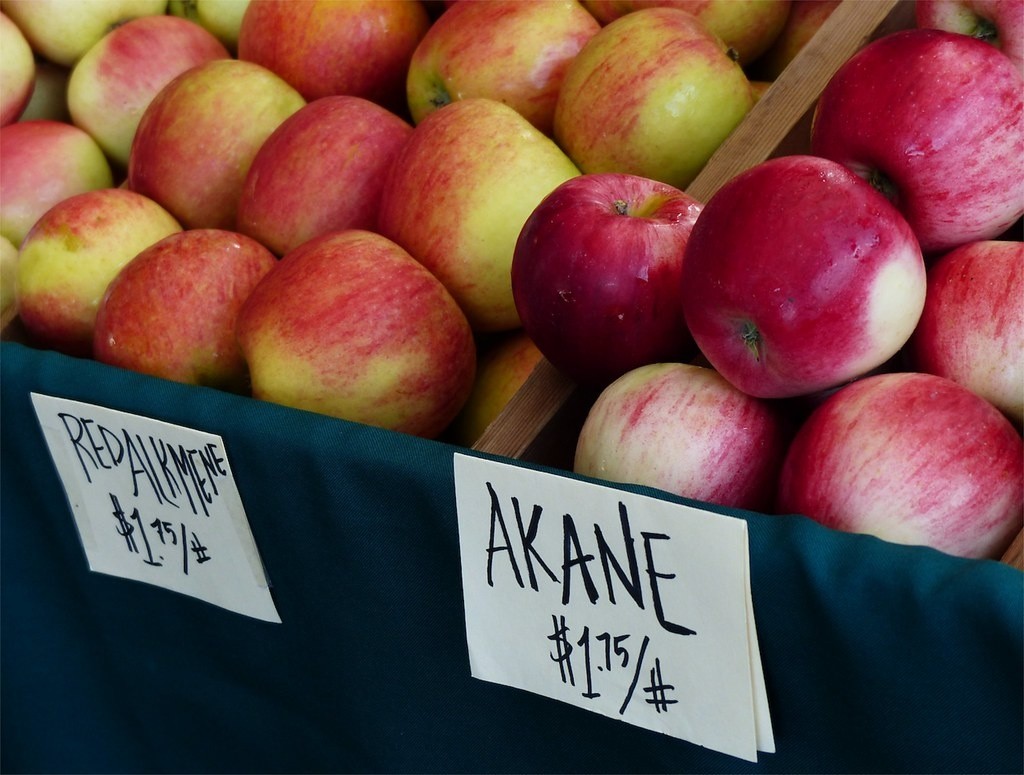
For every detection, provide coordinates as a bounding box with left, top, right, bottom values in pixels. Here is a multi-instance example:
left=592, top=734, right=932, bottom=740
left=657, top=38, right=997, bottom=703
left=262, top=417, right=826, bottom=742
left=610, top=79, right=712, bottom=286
left=0, top=0, right=1024, bottom=568
